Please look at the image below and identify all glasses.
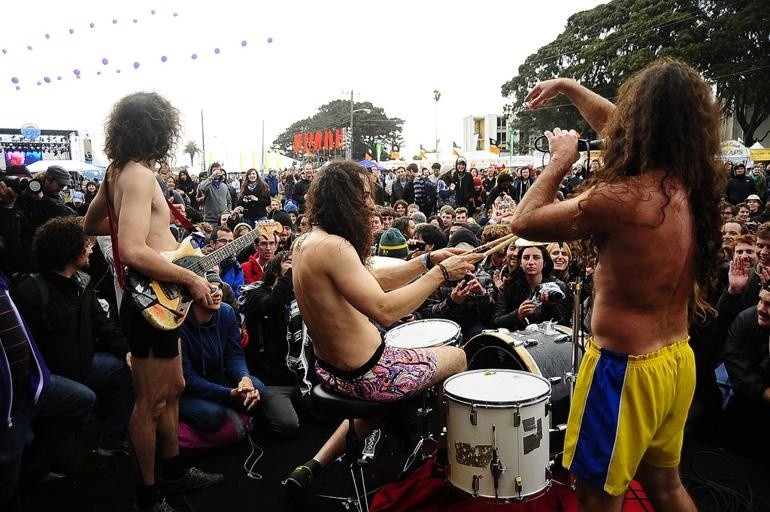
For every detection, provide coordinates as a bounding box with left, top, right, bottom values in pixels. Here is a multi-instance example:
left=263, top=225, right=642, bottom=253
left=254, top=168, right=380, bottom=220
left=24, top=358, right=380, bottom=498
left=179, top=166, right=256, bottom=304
left=257, top=243, right=276, bottom=248
left=216, top=239, right=234, bottom=243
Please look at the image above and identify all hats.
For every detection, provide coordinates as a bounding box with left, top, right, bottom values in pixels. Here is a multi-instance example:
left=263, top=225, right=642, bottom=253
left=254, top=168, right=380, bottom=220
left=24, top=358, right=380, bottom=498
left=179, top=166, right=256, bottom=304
left=285, top=203, right=299, bottom=216
left=206, top=270, right=224, bottom=288
left=473, top=176, right=483, bottom=186
left=734, top=164, right=745, bottom=168
left=74, top=192, right=85, bottom=203
left=378, top=227, right=410, bottom=258
left=446, top=227, right=479, bottom=248
left=274, top=210, right=293, bottom=227
left=457, top=157, right=467, bottom=167
left=45, top=166, right=72, bottom=186
left=744, top=194, right=762, bottom=203
left=440, top=163, right=454, bottom=175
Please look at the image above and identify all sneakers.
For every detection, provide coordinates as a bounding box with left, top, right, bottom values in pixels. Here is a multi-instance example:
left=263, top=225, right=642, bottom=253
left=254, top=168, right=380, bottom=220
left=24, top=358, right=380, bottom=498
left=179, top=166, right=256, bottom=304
left=175, top=467, right=224, bottom=494
left=281, top=468, right=312, bottom=498
left=92, top=443, right=131, bottom=457
left=41, top=472, right=77, bottom=487
left=134, top=497, right=175, bottom=512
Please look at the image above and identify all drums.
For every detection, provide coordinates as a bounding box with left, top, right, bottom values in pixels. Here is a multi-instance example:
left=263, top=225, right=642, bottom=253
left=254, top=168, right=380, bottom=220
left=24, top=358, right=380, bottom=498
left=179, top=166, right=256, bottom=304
left=286, top=299, right=307, bottom=370
left=443, top=369, right=553, bottom=504
left=462, top=325, right=592, bottom=456
left=384, top=318, right=461, bottom=350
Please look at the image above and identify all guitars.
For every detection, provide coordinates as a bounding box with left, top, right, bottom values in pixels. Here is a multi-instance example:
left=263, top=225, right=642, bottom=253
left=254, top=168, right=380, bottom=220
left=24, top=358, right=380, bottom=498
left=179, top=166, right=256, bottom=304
left=123, top=218, right=283, bottom=330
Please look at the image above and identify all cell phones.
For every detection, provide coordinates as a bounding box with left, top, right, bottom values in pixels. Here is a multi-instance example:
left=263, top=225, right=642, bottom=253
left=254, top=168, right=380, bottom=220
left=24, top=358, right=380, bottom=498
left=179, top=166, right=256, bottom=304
left=458, top=275, right=474, bottom=296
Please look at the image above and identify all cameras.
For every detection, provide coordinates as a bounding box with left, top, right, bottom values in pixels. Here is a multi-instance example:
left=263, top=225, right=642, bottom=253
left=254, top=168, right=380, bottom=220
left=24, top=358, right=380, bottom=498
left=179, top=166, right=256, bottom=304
left=534, top=284, right=564, bottom=304
left=0, top=171, right=28, bottom=192
left=29, top=179, right=42, bottom=192
left=216, top=175, right=224, bottom=181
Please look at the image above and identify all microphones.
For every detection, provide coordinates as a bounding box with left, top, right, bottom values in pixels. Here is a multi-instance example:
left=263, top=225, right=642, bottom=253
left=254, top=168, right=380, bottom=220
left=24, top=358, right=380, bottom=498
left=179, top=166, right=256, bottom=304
left=534, top=137, right=610, bottom=152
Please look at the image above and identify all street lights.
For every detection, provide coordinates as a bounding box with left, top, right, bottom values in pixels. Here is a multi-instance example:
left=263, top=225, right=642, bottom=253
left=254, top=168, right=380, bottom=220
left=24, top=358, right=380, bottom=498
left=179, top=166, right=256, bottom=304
left=349, top=107, right=372, bottom=159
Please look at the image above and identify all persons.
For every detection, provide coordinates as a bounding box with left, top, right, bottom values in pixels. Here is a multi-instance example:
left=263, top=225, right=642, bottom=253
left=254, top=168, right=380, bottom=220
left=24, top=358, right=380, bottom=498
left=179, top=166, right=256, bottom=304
left=220, top=169, right=249, bottom=208
left=573, top=236, right=600, bottom=335
left=201, top=162, right=232, bottom=228
left=266, top=164, right=314, bottom=212
left=279, top=158, right=467, bottom=499
left=0, top=275, right=97, bottom=512
left=83, top=92, right=225, bottom=512
left=552, top=160, right=603, bottom=206
left=709, top=156, right=769, bottom=328
left=546, top=242, right=577, bottom=298
left=420, top=228, right=496, bottom=341
left=158, top=168, right=206, bottom=229
left=365, top=163, right=446, bottom=215
left=9, top=215, right=137, bottom=482
left=449, top=159, right=537, bottom=231
left=243, top=250, right=320, bottom=441
left=725, top=281, right=770, bottom=483
left=178, top=271, right=267, bottom=470
left=178, top=206, right=311, bottom=294
left=0, top=164, right=100, bottom=268
left=364, top=200, right=466, bottom=262
left=509, top=59, right=725, bottom=512
left=496, top=245, right=573, bottom=333
left=238, top=168, right=270, bottom=225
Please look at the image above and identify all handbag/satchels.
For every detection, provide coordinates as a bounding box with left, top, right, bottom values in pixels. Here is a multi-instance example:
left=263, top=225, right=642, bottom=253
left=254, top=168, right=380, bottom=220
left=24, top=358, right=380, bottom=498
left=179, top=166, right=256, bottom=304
left=437, top=181, right=456, bottom=208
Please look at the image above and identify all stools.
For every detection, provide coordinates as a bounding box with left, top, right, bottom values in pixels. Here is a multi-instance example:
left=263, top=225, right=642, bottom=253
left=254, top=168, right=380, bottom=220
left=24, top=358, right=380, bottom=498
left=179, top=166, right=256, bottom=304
left=313, top=382, right=392, bottom=512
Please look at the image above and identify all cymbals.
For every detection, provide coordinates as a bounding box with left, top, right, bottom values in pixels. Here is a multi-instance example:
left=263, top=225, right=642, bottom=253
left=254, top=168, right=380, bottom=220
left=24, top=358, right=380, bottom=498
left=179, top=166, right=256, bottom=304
left=515, top=239, right=549, bottom=247
